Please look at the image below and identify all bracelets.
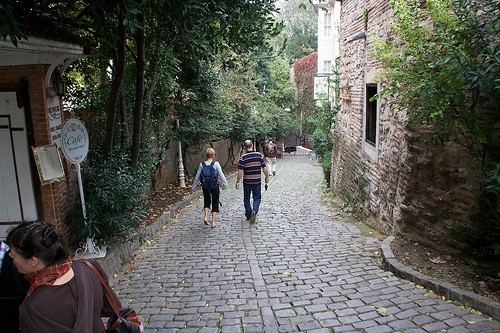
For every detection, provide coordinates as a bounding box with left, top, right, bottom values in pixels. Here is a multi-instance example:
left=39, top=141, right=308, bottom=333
left=237, top=180, right=239, bottom=183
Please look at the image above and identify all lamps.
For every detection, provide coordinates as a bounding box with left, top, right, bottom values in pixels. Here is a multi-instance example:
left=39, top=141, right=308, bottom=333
left=51, top=68, right=71, bottom=98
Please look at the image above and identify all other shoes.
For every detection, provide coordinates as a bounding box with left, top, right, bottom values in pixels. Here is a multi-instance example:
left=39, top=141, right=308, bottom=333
left=249, top=210, right=257, bottom=224
left=211, top=222, right=216, bottom=228
left=203, top=218, right=209, bottom=225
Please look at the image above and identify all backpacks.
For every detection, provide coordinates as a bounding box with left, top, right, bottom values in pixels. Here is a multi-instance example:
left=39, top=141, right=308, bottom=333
left=199, top=160, right=219, bottom=190
left=79, top=259, right=146, bottom=333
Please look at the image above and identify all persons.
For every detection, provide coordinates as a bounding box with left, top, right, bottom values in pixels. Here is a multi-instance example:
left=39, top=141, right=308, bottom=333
left=191, top=148, right=227, bottom=228
left=236, top=140, right=270, bottom=223
left=261, top=137, right=283, bottom=176
left=6, top=220, right=122, bottom=333
left=301, top=139, right=308, bottom=149
